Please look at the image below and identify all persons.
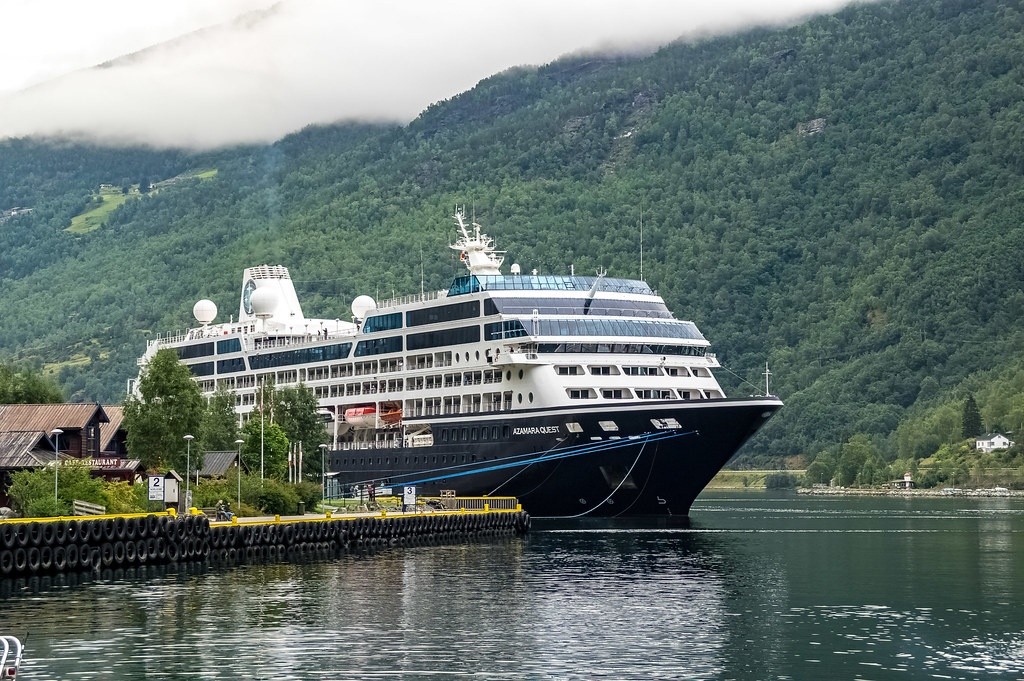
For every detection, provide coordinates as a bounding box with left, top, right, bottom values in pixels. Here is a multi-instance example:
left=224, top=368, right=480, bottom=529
left=317, top=328, right=328, bottom=340
left=349, top=484, right=359, bottom=497
left=367, top=483, right=372, bottom=500
left=356, top=323, right=360, bottom=331
left=215, top=500, right=234, bottom=522
left=401, top=495, right=407, bottom=512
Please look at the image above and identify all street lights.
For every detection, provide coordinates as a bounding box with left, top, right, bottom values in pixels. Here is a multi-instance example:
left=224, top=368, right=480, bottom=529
left=318, top=442, right=327, bottom=513
left=183, top=434, right=194, bottom=514
left=235, top=439, right=246, bottom=510
left=51, top=429, right=64, bottom=506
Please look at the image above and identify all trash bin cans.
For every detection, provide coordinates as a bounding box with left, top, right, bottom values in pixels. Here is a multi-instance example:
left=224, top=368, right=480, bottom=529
left=299, top=501, right=305, bottom=516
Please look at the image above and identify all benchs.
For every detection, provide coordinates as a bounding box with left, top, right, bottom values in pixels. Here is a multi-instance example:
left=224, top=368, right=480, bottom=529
left=215, top=513, right=229, bottom=521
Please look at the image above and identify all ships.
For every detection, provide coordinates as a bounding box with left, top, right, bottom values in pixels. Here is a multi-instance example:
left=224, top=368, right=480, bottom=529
left=124, top=203, right=784, bottom=533
